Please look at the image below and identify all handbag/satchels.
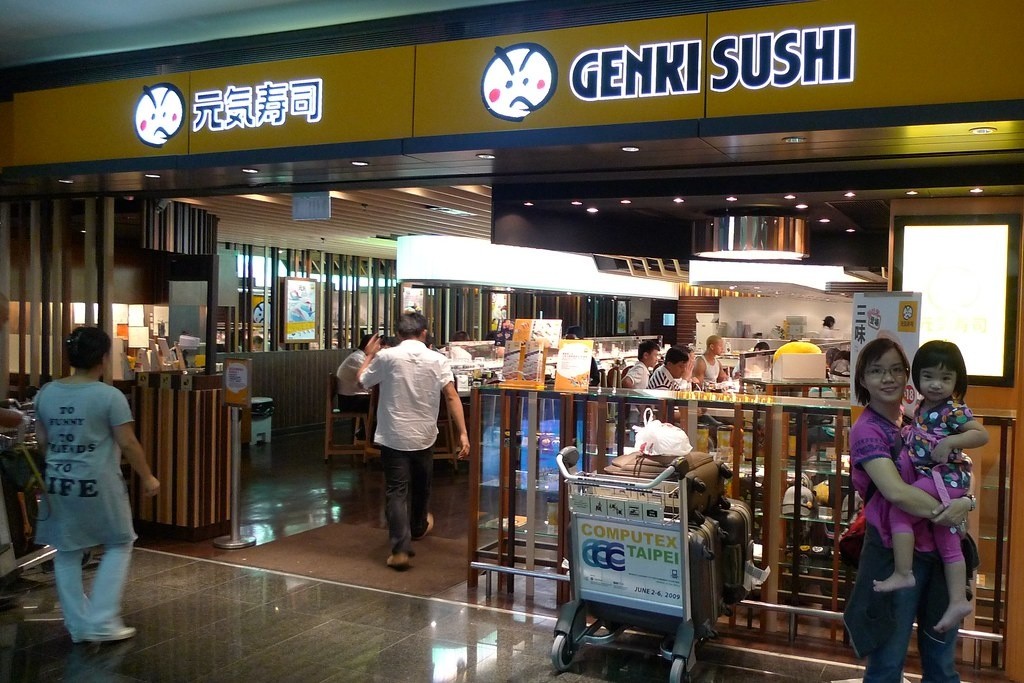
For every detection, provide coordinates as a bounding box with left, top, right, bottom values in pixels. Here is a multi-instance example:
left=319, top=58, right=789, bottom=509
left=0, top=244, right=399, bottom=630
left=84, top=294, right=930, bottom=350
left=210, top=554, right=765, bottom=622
left=632, top=408, right=693, bottom=457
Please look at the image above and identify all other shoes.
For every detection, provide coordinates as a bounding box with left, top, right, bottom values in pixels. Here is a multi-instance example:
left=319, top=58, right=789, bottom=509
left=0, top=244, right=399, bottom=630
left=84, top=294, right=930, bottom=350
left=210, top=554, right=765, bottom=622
left=386, top=551, right=410, bottom=571
left=83, top=622, right=136, bottom=642
left=412, top=513, right=435, bottom=540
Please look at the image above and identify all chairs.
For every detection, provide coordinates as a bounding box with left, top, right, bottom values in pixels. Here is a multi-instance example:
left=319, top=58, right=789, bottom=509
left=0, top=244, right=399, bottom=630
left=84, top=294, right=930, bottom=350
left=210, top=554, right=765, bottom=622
left=323, top=372, right=367, bottom=461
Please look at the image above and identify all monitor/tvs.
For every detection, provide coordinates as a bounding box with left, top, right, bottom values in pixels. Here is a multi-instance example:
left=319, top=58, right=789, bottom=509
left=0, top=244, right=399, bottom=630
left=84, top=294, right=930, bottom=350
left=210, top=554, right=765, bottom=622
left=663, top=313, right=675, bottom=326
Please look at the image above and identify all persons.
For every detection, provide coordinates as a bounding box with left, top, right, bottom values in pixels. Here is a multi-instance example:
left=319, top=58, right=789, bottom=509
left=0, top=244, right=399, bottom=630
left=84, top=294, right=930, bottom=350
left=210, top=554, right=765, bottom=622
left=693, top=335, right=727, bottom=383
left=33, top=326, right=159, bottom=642
left=357, top=312, right=470, bottom=568
left=566, top=326, right=600, bottom=387
left=732, top=342, right=770, bottom=379
left=253, top=336, right=282, bottom=352
left=647, top=343, right=700, bottom=424
left=851, top=338, right=975, bottom=683
left=621, top=341, right=660, bottom=422
left=337, top=334, right=373, bottom=412
left=873, top=341, right=990, bottom=634
left=440, top=331, right=472, bottom=360
left=821, top=316, right=835, bottom=337
left=806, top=387, right=837, bottom=448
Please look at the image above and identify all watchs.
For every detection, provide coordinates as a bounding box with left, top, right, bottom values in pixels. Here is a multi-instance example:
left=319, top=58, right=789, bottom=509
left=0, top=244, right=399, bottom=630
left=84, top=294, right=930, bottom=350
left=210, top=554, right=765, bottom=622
left=964, top=494, right=977, bottom=512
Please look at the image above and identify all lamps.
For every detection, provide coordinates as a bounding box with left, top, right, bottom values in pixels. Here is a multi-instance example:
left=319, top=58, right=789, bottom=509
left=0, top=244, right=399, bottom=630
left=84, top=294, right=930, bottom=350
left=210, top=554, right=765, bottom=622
left=691, top=214, right=812, bottom=259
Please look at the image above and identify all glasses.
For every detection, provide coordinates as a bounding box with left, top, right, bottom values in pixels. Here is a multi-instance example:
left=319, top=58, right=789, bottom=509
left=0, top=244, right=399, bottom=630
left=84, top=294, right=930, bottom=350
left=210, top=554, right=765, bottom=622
left=865, top=366, right=909, bottom=378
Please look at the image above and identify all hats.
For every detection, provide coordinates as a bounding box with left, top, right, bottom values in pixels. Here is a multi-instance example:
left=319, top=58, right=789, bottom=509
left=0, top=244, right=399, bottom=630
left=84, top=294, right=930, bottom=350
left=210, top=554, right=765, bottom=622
left=783, top=484, right=815, bottom=516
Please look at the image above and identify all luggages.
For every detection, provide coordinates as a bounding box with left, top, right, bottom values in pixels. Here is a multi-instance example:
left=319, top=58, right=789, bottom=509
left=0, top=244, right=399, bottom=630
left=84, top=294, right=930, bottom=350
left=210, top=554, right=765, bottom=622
left=604, top=454, right=753, bottom=643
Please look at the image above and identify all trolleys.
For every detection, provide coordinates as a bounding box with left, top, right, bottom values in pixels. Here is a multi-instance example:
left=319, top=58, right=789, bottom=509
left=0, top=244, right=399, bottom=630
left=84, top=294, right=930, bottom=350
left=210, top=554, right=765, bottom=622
left=549, top=441, right=719, bottom=683
